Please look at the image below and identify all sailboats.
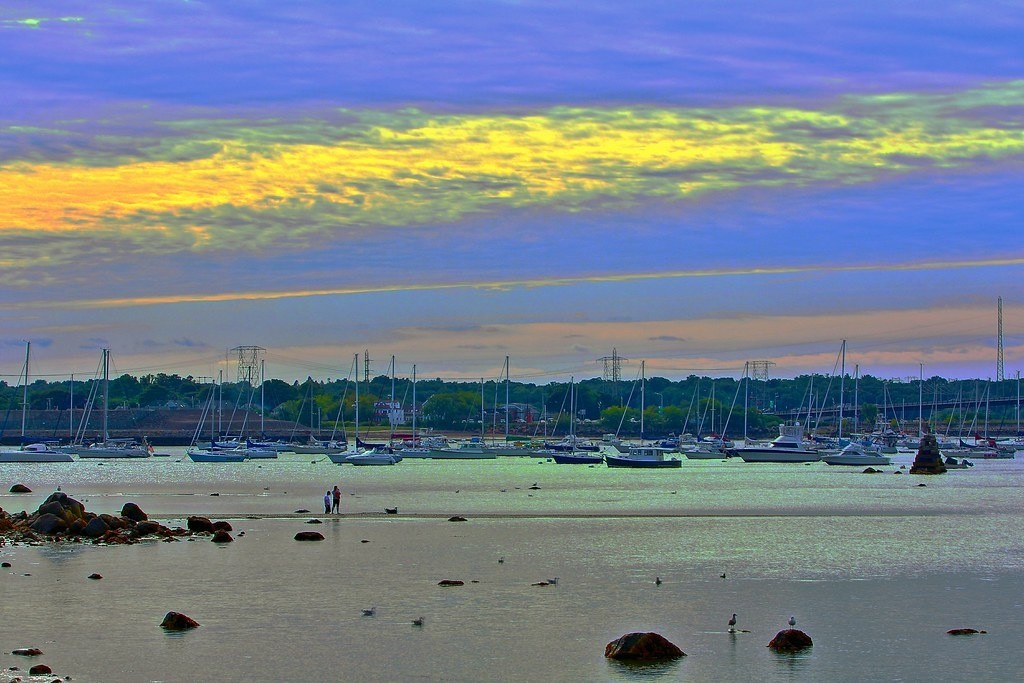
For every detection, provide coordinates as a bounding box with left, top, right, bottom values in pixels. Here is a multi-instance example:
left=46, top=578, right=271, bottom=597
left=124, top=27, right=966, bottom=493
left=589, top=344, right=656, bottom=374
left=552, top=383, right=603, bottom=464
left=345, top=355, right=403, bottom=466
left=0, top=339, right=74, bottom=463
left=392, top=364, right=569, bottom=459
left=24, top=347, right=151, bottom=458
left=324, top=353, right=363, bottom=464
left=195, top=360, right=347, bottom=459
left=186, top=380, right=246, bottom=462
left=679, top=339, right=1024, bottom=458
left=610, top=360, right=653, bottom=453
left=544, top=376, right=600, bottom=452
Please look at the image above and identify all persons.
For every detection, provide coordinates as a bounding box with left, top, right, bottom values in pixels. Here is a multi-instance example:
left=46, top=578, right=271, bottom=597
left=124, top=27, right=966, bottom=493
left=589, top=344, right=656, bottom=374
left=332, top=485, right=341, bottom=514
left=323, top=490, right=331, bottom=514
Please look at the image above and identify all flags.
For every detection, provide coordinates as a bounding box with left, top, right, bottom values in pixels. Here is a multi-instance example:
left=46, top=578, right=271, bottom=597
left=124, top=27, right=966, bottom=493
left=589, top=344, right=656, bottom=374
left=526, top=413, right=534, bottom=424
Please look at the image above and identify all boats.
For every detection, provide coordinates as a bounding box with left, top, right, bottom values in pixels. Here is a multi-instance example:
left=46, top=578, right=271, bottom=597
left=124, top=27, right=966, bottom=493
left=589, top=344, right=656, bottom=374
left=605, top=444, right=681, bottom=468
left=733, top=419, right=821, bottom=463
left=820, top=443, right=891, bottom=466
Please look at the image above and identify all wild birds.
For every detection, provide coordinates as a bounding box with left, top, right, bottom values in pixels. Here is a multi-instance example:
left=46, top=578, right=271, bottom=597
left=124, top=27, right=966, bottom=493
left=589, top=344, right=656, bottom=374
left=533, top=482, right=537, bottom=486
left=547, top=577, right=557, bottom=584
left=57, top=486, right=62, bottom=491
left=728, top=614, right=738, bottom=627
left=411, top=617, right=424, bottom=626
left=498, top=557, right=504, bottom=565
left=362, top=607, right=378, bottom=616
left=788, top=616, right=796, bottom=630
left=655, top=576, right=662, bottom=585
left=720, top=573, right=726, bottom=578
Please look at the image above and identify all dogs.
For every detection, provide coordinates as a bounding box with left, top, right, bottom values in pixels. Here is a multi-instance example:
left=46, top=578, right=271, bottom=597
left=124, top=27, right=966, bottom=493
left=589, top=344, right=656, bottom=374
left=384, top=507, right=397, bottom=515
